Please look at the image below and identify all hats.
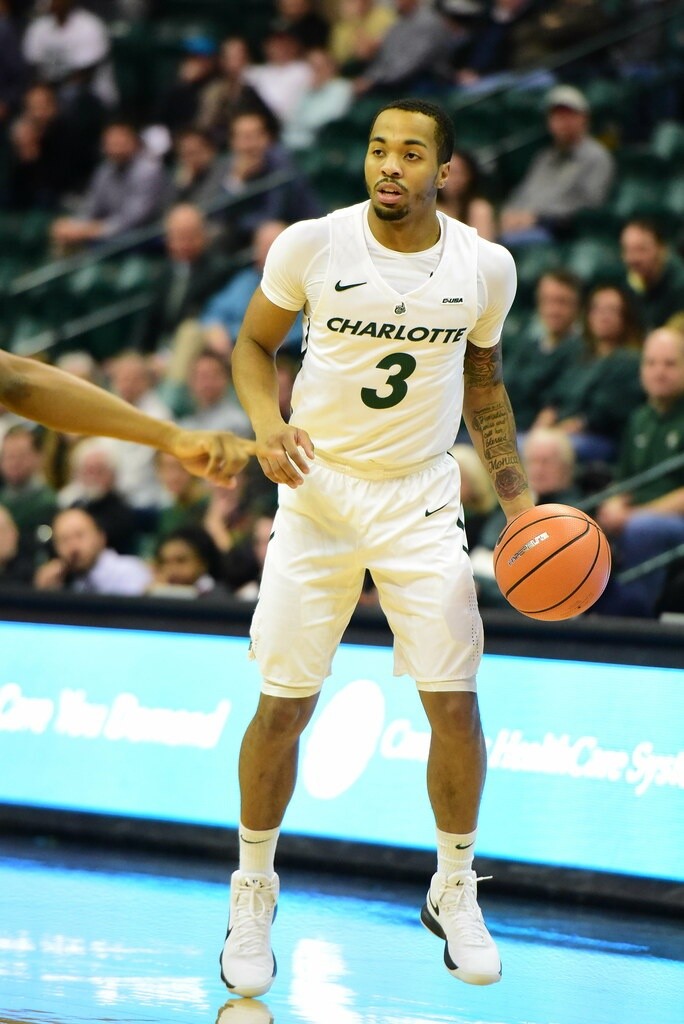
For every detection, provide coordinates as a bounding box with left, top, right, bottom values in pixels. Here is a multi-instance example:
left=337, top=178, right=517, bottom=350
left=543, top=86, right=590, bottom=114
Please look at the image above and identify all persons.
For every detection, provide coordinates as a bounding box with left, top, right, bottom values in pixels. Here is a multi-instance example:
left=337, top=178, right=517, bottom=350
left=220, top=101, right=536, bottom=996
left=0, top=0, right=684, bottom=619
left=0, top=347, right=257, bottom=489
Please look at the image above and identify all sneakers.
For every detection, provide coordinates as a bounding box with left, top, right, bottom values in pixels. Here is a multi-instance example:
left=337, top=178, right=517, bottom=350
left=420, top=871, right=502, bottom=984
left=223, top=870, right=280, bottom=997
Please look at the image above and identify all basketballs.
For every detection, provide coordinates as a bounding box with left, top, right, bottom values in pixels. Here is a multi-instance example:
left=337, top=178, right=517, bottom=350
left=492, top=501, right=615, bottom=623
